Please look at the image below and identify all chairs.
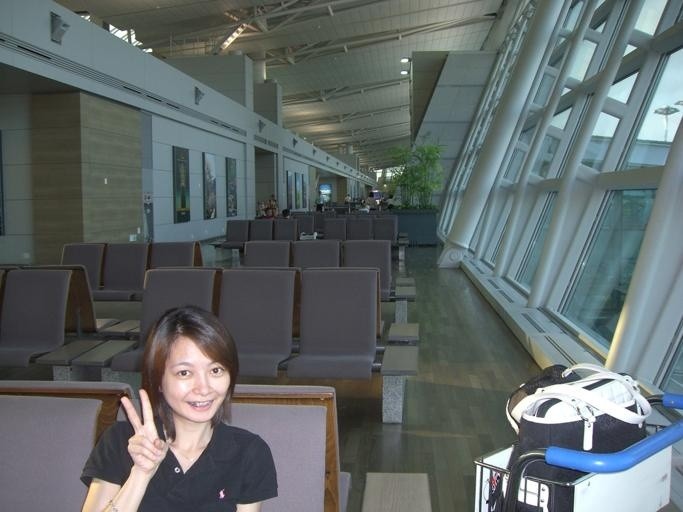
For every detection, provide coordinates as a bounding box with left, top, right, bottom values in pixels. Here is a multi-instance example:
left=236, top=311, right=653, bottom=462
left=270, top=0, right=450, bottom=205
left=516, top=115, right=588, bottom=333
left=0, top=206, right=421, bottom=511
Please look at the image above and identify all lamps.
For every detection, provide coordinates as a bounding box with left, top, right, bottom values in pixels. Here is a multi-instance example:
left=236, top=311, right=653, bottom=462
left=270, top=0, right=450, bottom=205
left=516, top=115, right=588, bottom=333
left=399, top=70, right=410, bottom=76
left=399, top=56, right=409, bottom=63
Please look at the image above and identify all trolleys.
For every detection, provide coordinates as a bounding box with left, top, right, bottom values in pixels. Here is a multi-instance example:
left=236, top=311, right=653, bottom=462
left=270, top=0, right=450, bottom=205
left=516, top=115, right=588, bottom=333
left=471, top=394, right=682, bottom=512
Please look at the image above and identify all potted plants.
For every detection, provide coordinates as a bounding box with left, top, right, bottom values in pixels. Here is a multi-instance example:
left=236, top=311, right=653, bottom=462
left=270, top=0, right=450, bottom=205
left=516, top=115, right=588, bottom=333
left=385, top=131, right=451, bottom=246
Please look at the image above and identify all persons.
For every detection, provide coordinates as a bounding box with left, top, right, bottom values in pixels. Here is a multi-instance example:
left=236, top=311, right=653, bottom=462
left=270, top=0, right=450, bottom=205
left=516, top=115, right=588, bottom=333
left=282, top=209, right=290, bottom=218
left=81, top=305, right=278, bottom=511
left=382, top=195, right=396, bottom=205
left=265, top=208, right=273, bottom=216
left=315, top=191, right=324, bottom=213
left=345, top=194, right=351, bottom=202
left=366, top=192, right=377, bottom=210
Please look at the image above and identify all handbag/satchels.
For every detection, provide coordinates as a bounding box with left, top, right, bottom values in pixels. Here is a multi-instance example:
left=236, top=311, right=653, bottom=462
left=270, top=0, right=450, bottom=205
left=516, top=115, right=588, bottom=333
left=484, top=363, right=653, bottom=512
